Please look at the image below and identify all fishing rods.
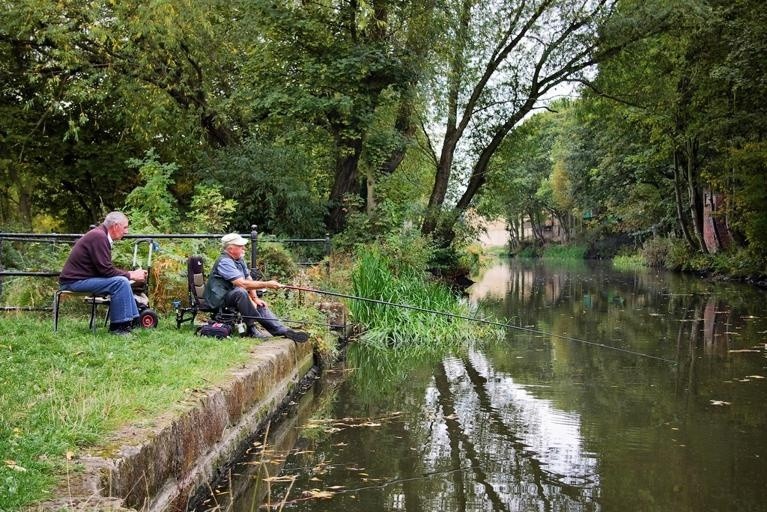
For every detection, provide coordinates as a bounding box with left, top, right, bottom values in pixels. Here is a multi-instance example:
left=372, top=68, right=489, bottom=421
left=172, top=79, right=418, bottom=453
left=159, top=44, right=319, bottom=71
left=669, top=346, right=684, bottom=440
left=222, top=310, right=342, bottom=328
left=284, top=280, right=679, bottom=368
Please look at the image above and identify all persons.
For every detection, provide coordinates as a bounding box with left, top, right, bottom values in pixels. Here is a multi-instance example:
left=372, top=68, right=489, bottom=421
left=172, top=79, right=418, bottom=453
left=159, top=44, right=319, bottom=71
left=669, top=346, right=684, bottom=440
left=59, top=211, right=149, bottom=336
left=202, top=229, right=308, bottom=343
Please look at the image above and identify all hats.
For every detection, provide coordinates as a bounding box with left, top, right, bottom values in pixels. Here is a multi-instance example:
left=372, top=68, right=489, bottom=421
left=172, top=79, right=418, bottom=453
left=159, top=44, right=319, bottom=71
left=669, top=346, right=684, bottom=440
left=221, top=233, right=248, bottom=247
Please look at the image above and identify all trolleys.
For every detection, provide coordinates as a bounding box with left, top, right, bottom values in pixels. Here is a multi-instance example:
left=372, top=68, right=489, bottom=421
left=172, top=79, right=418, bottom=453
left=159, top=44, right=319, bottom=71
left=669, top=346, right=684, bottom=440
left=84, top=237, right=159, bottom=331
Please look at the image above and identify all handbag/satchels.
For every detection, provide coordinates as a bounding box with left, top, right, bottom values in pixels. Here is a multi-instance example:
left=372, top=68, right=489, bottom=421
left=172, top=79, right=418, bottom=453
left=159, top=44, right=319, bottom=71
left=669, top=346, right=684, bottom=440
left=194, top=324, right=232, bottom=339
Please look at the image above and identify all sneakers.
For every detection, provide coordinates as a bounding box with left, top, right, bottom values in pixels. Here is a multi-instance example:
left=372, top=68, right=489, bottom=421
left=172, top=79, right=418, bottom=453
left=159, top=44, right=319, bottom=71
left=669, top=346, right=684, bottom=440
left=108, top=326, right=134, bottom=336
left=283, top=329, right=308, bottom=343
left=247, top=326, right=267, bottom=339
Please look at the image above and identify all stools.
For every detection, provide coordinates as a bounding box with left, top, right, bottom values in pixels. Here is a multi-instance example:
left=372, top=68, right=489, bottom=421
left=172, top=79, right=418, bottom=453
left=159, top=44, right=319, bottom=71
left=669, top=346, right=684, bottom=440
left=52, top=290, right=111, bottom=330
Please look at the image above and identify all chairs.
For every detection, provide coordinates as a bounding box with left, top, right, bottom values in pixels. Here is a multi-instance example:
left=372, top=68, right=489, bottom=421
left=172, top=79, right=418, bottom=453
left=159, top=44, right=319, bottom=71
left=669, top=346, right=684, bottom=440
left=176, top=255, right=246, bottom=337
